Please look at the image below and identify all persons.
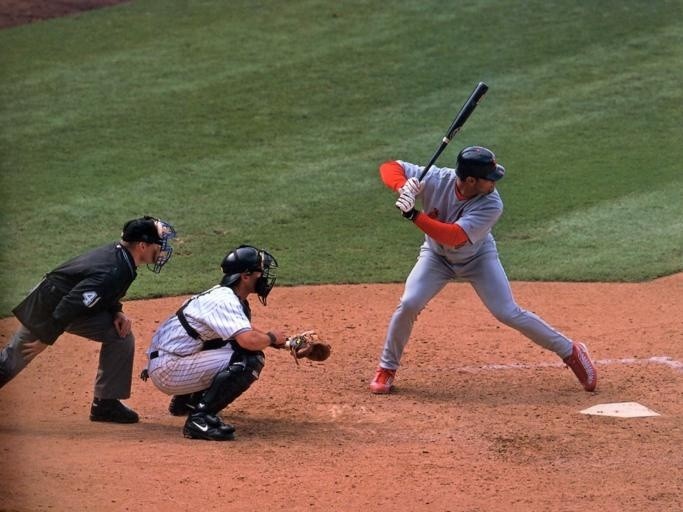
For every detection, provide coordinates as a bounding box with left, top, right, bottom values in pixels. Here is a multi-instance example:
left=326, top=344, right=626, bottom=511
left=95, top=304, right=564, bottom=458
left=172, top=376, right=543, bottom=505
left=370, top=145, right=598, bottom=393
left=0, top=216, right=177, bottom=423
left=147, top=245, right=289, bottom=441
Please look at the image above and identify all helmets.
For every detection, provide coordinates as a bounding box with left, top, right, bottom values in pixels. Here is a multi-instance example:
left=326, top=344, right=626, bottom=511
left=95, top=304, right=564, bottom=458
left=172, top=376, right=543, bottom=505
left=454, top=146, right=504, bottom=181
left=219, top=245, right=277, bottom=306
left=122, top=215, right=175, bottom=274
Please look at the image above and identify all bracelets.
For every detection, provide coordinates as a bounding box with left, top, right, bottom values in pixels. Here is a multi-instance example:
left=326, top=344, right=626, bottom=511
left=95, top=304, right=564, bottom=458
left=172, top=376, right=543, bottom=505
left=266, top=332, right=277, bottom=343
left=402, top=208, right=419, bottom=223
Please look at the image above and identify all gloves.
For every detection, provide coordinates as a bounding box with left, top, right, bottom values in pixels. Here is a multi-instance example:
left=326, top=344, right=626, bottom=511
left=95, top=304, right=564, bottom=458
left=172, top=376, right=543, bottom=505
left=401, top=177, right=425, bottom=196
left=395, top=188, right=415, bottom=220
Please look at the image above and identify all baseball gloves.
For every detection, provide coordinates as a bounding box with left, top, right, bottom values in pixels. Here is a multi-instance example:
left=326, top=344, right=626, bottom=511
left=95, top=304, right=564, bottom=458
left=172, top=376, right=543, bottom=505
left=285, top=329, right=329, bottom=361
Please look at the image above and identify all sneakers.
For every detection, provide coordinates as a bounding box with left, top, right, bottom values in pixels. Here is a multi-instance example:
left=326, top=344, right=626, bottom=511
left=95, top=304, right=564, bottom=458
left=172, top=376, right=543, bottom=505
left=562, top=342, right=596, bottom=391
left=168, top=396, right=198, bottom=416
left=183, top=412, right=235, bottom=439
left=89, top=399, right=137, bottom=423
left=370, top=367, right=395, bottom=394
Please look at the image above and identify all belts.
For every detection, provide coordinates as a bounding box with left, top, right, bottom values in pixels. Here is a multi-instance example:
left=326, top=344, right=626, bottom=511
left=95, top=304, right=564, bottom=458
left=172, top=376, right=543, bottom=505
left=150, top=351, right=158, bottom=359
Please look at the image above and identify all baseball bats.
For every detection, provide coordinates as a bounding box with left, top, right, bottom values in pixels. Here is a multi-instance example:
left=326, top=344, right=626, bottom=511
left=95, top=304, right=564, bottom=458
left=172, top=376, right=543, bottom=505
left=419, top=82, right=488, bottom=183
